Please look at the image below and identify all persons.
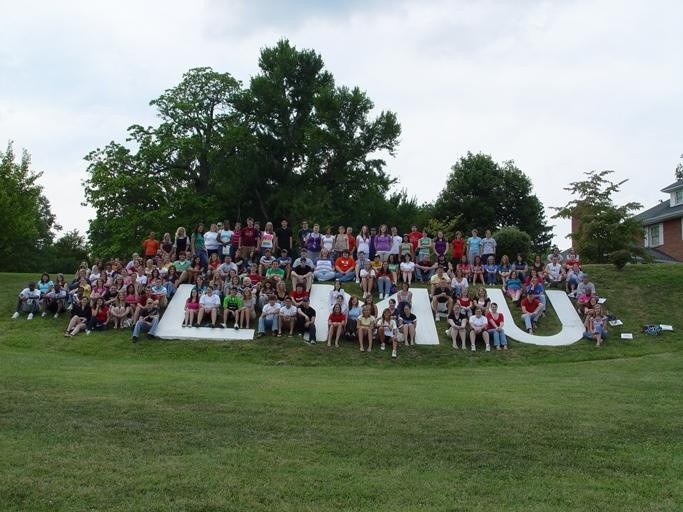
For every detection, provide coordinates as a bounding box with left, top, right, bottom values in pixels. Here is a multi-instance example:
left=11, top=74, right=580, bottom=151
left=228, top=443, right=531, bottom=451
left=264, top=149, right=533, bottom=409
left=327, top=279, right=417, bottom=357
left=133, top=224, right=208, bottom=343
left=432, top=279, right=508, bottom=351
left=12, top=273, right=68, bottom=319
left=65, top=253, right=147, bottom=336
left=305, top=223, right=496, bottom=299
left=498, top=247, right=609, bottom=347
left=183, top=219, right=316, bottom=344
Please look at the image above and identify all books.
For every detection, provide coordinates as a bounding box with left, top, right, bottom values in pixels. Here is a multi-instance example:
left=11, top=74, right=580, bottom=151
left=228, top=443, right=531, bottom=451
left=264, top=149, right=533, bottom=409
left=659, top=324, right=673, bottom=331
left=608, top=319, right=623, bottom=326
left=620, top=333, right=633, bottom=339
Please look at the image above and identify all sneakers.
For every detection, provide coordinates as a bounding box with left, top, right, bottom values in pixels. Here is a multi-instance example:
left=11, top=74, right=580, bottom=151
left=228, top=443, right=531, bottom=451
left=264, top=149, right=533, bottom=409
left=595, top=338, right=604, bottom=346
left=360, top=344, right=372, bottom=352
left=257, top=330, right=304, bottom=338
left=496, top=346, right=508, bottom=352
left=363, top=291, right=371, bottom=299
left=326, top=343, right=339, bottom=348
left=489, top=282, right=496, bottom=287
left=345, top=332, right=356, bottom=337
left=182, top=323, right=240, bottom=330
left=355, top=278, right=360, bottom=284
left=132, top=336, right=137, bottom=343
left=512, top=298, right=520, bottom=303
left=310, top=339, right=316, bottom=345
left=380, top=343, right=386, bottom=351
left=452, top=345, right=468, bottom=351
left=529, top=322, right=537, bottom=334
left=147, top=334, right=152, bottom=340
left=27, top=312, right=33, bottom=320
left=11, top=311, right=20, bottom=320
left=113, top=322, right=136, bottom=330
left=544, top=281, right=552, bottom=288
left=40, top=312, right=60, bottom=320
left=378, top=293, right=390, bottom=300
left=64, top=331, right=74, bottom=338
left=392, top=349, right=398, bottom=358
left=435, top=313, right=440, bottom=322
left=404, top=341, right=415, bottom=347
left=470, top=344, right=477, bottom=353
left=485, top=344, right=491, bottom=352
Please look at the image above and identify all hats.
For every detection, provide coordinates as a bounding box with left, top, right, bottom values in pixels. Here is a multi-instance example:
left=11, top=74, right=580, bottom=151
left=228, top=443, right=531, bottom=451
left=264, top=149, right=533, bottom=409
left=303, top=296, right=309, bottom=302
left=526, top=290, right=535, bottom=295
left=265, top=248, right=271, bottom=252
left=373, top=254, right=380, bottom=258
left=147, top=298, right=153, bottom=304
left=281, top=248, right=287, bottom=254
left=301, top=247, right=307, bottom=252
left=438, top=254, right=445, bottom=257
